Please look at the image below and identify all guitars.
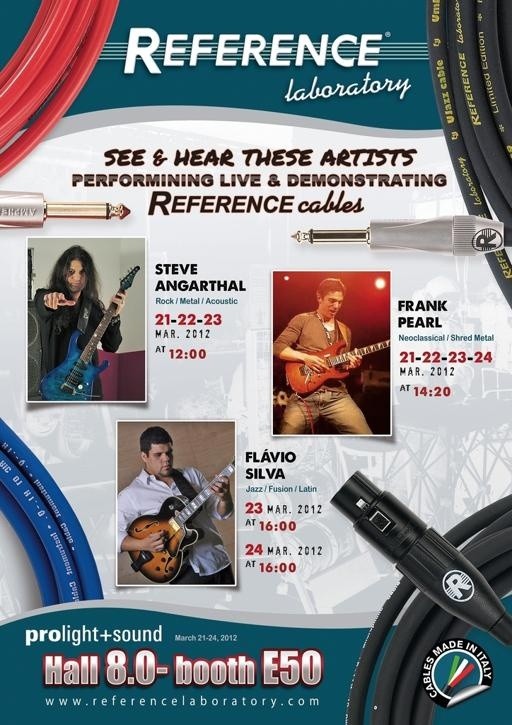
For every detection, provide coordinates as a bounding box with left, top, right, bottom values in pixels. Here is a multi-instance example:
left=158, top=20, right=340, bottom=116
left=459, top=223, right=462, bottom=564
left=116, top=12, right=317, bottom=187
left=285, top=340, right=390, bottom=395
left=39, top=265, right=141, bottom=401
left=128, top=458, right=235, bottom=584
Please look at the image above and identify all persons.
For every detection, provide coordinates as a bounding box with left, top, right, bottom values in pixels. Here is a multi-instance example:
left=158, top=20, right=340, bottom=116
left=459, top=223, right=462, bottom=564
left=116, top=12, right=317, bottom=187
left=31, top=242, right=128, bottom=401
left=116, top=423, right=236, bottom=585
left=272, top=277, right=375, bottom=435
